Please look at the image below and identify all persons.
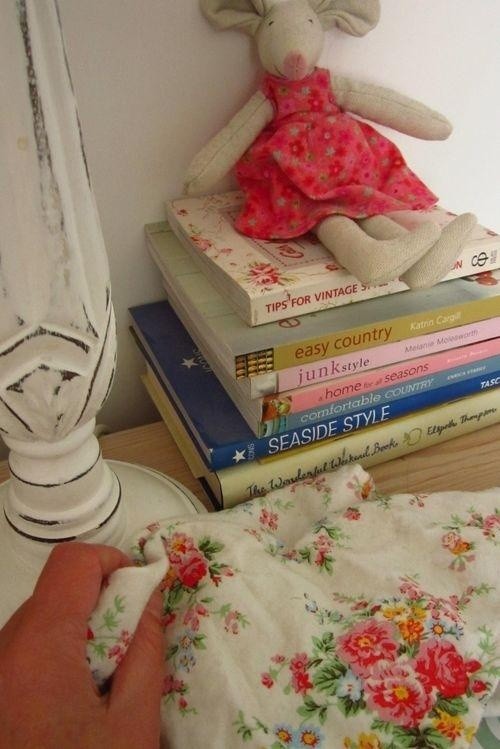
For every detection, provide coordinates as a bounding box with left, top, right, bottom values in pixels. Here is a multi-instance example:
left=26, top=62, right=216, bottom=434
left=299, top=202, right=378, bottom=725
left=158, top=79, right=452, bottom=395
left=1, top=540, right=166, bottom=748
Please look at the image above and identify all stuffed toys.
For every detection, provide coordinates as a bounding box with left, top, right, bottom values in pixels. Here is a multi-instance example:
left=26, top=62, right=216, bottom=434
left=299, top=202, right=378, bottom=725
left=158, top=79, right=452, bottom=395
left=184, top=1, right=479, bottom=292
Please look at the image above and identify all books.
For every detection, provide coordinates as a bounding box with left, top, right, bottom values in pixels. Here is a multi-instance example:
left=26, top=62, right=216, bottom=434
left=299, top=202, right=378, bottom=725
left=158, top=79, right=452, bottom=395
left=124, top=186, right=498, bottom=513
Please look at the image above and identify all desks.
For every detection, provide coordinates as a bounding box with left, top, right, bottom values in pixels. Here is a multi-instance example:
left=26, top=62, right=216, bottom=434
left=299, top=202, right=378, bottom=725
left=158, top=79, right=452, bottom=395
left=0, top=420, right=500, bottom=749
